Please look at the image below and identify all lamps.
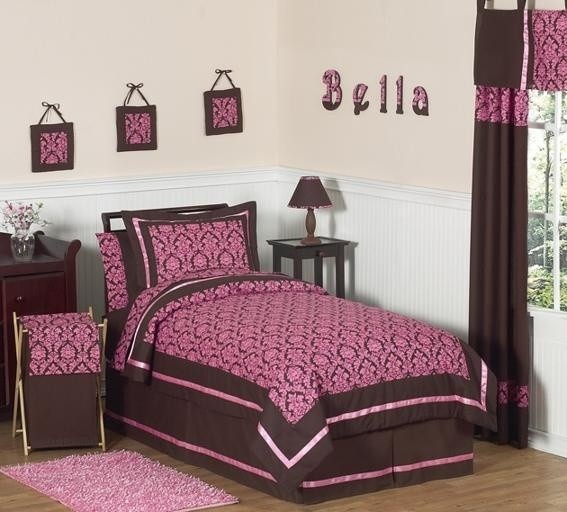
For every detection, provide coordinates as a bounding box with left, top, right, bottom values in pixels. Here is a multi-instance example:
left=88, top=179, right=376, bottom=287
left=287, top=176, right=332, bottom=245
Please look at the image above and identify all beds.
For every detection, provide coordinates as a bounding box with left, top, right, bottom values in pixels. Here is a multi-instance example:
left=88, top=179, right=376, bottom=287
left=102, top=203, right=497, bottom=506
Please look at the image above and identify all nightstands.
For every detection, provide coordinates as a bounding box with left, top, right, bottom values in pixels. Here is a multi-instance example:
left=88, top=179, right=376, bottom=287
left=1, top=232, right=81, bottom=413
left=266, top=237, right=349, bottom=299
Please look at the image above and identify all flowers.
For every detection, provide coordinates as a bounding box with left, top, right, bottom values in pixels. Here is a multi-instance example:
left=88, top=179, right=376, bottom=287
left=1, top=199, right=51, bottom=231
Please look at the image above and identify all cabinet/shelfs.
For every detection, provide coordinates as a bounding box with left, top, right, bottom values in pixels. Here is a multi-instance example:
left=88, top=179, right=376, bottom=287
left=10, top=306, right=110, bottom=455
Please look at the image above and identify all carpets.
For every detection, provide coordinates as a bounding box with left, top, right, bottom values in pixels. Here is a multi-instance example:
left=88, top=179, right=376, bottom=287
left=0, top=449, right=239, bottom=512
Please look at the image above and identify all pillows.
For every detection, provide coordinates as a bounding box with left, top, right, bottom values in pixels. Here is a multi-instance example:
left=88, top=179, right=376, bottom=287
left=122, top=201, right=261, bottom=288
left=95, top=230, right=138, bottom=313
left=138, top=214, right=255, bottom=290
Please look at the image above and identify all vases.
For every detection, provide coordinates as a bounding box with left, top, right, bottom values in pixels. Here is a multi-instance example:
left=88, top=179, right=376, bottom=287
left=11, top=226, right=34, bottom=261
